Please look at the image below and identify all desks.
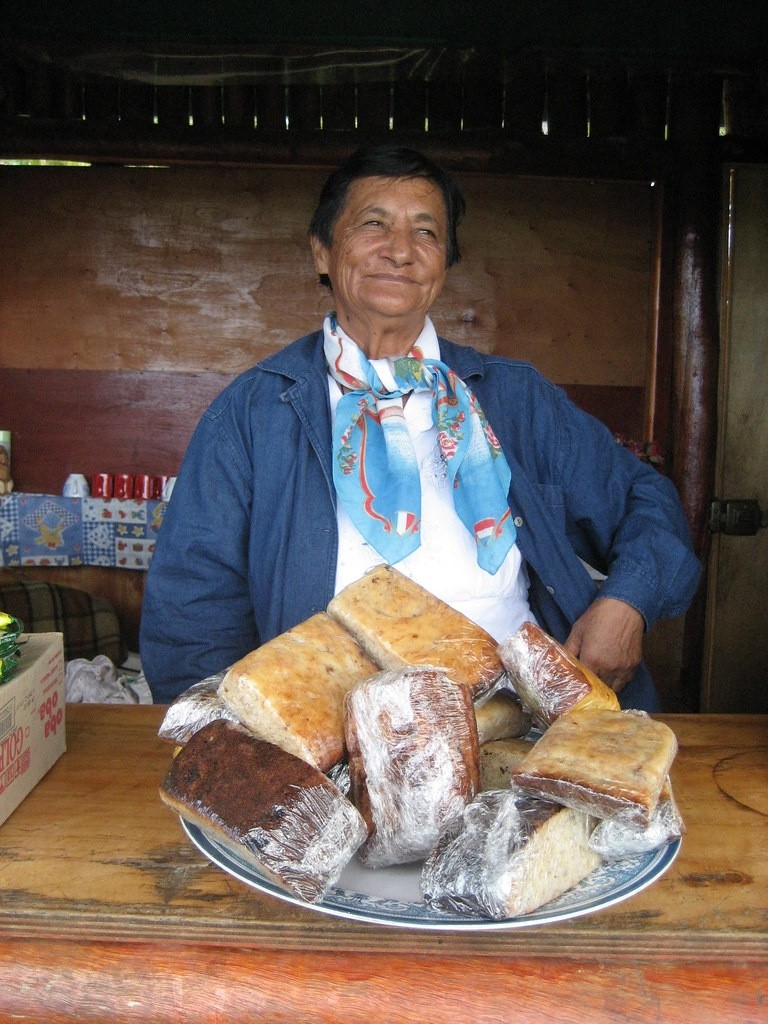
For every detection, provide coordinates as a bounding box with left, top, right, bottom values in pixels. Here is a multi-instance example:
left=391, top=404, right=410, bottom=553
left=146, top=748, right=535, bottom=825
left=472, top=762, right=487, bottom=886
left=0, top=703, right=768, bottom=1024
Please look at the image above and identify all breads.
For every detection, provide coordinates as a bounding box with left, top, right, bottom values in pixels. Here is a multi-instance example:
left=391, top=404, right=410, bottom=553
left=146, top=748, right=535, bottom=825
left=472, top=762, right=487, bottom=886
left=158, top=563, right=683, bottom=920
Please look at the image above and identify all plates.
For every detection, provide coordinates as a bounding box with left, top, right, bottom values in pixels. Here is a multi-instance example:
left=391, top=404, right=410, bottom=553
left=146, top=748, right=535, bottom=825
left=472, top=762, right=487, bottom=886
left=180, top=728, right=682, bottom=929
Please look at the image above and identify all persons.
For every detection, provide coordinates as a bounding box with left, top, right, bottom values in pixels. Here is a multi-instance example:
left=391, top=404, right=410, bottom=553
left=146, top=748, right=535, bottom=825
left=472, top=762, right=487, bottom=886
left=138, top=143, right=705, bottom=713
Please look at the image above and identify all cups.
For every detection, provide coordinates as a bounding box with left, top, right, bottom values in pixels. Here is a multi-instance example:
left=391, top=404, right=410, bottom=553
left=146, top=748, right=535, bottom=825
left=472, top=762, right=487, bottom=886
left=162, top=476, right=177, bottom=502
left=63, top=474, right=90, bottom=498
left=114, top=474, right=133, bottom=499
left=92, top=473, right=113, bottom=498
left=134, top=475, right=152, bottom=499
left=152, top=474, right=170, bottom=499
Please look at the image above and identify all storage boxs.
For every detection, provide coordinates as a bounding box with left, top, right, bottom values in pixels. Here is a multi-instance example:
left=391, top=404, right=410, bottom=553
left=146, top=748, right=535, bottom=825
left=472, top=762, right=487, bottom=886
left=0, top=632, right=67, bottom=828
